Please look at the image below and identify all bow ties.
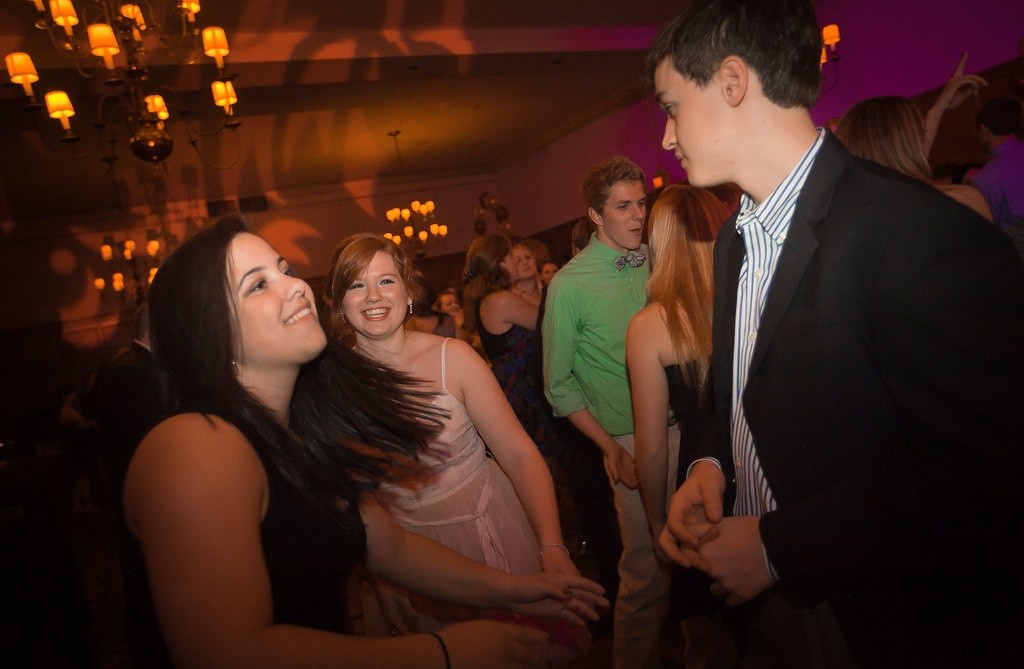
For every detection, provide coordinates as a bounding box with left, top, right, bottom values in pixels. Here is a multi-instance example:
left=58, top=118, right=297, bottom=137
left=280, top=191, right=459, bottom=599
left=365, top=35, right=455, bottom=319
left=616, top=251, right=645, bottom=270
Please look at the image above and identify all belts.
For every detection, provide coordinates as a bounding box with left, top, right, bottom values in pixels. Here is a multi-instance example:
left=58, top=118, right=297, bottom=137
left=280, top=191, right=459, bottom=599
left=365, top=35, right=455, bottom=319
left=667, top=417, right=678, bottom=426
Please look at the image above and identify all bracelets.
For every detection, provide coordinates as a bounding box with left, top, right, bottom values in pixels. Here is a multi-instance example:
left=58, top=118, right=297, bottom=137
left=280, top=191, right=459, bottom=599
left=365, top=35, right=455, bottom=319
left=538, top=541, right=570, bottom=557
left=425, top=629, right=453, bottom=669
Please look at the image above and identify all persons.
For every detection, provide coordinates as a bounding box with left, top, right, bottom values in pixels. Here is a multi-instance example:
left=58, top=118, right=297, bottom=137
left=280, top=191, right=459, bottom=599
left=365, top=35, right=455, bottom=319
left=121, top=212, right=610, bottom=669
left=100, top=299, right=150, bottom=668
left=428, top=0, right=1024, bottom=669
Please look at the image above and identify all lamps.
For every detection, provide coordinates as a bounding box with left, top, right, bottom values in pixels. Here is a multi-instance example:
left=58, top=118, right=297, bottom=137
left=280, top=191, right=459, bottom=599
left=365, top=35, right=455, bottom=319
left=383, top=130, right=449, bottom=263
left=820, top=23, right=841, bottom=62
left=93, top=235, right=161, bottom=310
left=0, top=0, right=242, bottom=172
left=652, top=169, right=667, bottom=188
